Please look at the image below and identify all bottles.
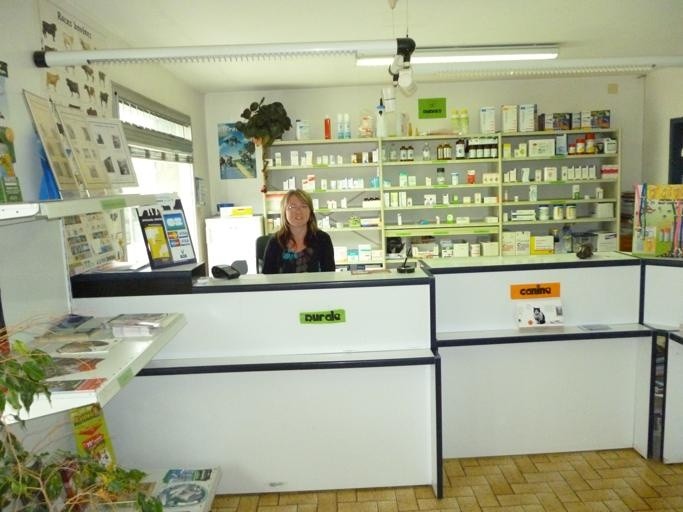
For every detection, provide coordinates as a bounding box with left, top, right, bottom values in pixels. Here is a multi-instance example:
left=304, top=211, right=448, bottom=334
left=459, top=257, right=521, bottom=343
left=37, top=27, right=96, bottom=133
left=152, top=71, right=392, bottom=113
left=453, top=110, right=469, bottom=134
left=381, top=143, right=415, bottom=161
left=436, top=168, right=445, bottom=185
left=569, top=134, right=599, bottom=154
left=437, top=142, right=452, bottom=160
left=456, top=138, right=466, bottom=158
left=422, top=142, right=432, bottom=160
left=470, top=139, right=499, bottom=159
left=539, top=205, right=577, bottom=223
left=324, top=115, right=332, bottom=140
left=336, top=114, right=351, bottom=140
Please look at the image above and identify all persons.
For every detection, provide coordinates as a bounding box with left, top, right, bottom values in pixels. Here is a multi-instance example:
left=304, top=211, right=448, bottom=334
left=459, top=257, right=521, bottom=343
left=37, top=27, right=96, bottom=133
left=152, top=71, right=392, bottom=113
left=260, top=190, right=335, bottom=274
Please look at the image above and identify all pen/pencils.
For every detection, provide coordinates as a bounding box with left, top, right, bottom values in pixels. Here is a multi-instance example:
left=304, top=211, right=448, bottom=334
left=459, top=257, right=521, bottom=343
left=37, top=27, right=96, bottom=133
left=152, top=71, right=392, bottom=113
left=404, top=247, right=411, bottom=268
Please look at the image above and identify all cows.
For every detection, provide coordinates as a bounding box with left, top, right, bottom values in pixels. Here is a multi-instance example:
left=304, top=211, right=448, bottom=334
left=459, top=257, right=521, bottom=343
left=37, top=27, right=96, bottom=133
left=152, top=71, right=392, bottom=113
left=40, top=19, right=110, bottom=106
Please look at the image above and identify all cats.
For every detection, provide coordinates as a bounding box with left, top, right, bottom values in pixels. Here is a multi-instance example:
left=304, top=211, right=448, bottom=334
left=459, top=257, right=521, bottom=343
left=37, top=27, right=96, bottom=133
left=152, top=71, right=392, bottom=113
left=533, top=307, right=546, bottom=324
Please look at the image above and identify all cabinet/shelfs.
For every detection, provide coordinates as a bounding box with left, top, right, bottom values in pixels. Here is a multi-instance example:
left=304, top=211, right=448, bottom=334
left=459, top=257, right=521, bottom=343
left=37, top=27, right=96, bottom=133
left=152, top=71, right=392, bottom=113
left=501, top=130, right=620, bottom=255
left=255, top=138, right=382, bottom=271
left=381, top=134, right=501, bottom=264
left=69, top=250, right=682, bottom=500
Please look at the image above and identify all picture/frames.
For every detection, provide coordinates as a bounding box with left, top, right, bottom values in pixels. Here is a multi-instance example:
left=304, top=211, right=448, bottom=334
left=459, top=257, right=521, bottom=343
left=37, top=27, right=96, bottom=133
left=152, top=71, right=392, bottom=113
left=89, top=116, right=140, bottom=188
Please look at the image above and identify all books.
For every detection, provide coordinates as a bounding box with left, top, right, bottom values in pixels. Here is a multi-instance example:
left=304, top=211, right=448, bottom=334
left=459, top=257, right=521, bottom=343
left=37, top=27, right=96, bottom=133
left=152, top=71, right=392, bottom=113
left=20, top=312, right=169, bottom=390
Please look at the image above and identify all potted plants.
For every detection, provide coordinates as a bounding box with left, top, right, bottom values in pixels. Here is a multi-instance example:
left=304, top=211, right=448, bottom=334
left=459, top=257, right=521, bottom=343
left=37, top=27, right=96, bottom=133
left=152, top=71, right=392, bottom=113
left=236, top=97, right=291, bottom=145
